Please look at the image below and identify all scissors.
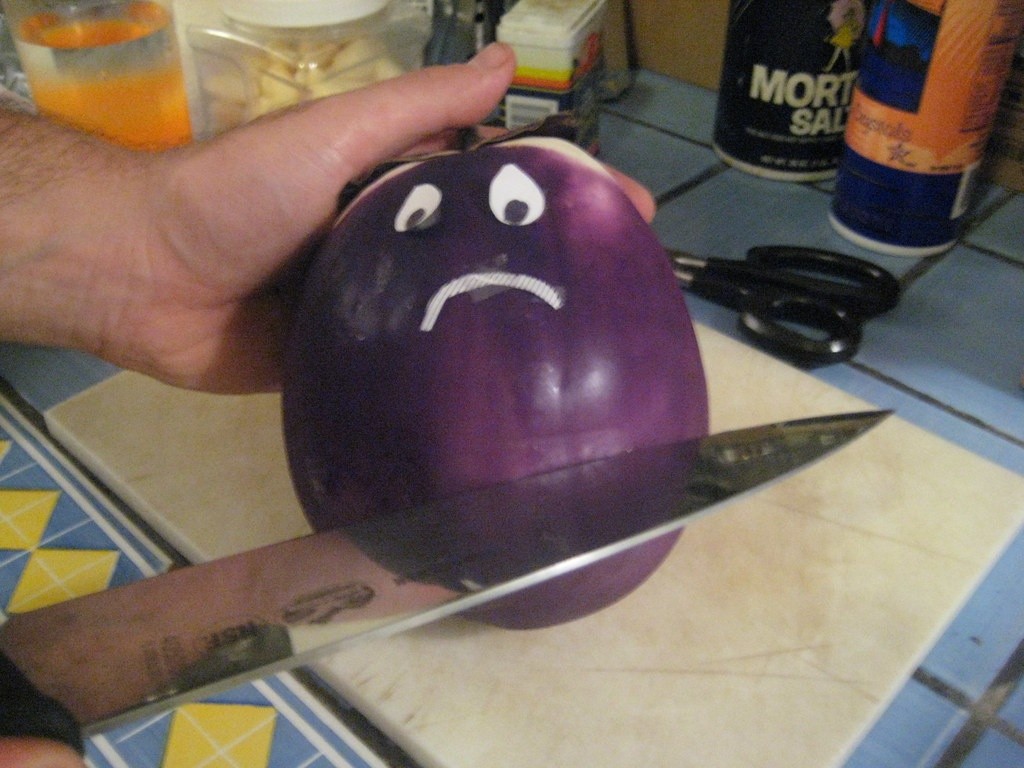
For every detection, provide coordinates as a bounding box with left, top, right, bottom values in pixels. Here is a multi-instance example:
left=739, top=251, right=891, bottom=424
left=666, top=245, right=899, bottom=363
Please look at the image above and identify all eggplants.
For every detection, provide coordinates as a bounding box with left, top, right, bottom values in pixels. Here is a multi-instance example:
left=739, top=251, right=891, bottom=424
left=278, top=115, right=709, bottom=629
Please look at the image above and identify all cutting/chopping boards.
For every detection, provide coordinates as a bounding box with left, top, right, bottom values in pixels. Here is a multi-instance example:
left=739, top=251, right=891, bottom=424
left=41, top=317, right=1024, bottom=768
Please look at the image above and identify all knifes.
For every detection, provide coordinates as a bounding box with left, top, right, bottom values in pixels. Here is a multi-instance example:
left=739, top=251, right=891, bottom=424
left=0, top=410, right=898, bottom=755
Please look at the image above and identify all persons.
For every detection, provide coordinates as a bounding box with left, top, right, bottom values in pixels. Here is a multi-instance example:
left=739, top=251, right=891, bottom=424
left=0, top=41, right=655, bottom=768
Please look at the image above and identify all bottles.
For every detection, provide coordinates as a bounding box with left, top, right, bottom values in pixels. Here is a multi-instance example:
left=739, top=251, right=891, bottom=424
left=827, top=2, right=1024, bottom=257
left=183, top=0, right=431, bottom=141
left=711, top=0, right=871, bottom=182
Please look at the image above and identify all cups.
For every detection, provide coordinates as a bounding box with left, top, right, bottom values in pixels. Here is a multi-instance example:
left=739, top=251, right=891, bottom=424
left=0, top=0, right=193, bottom=154
